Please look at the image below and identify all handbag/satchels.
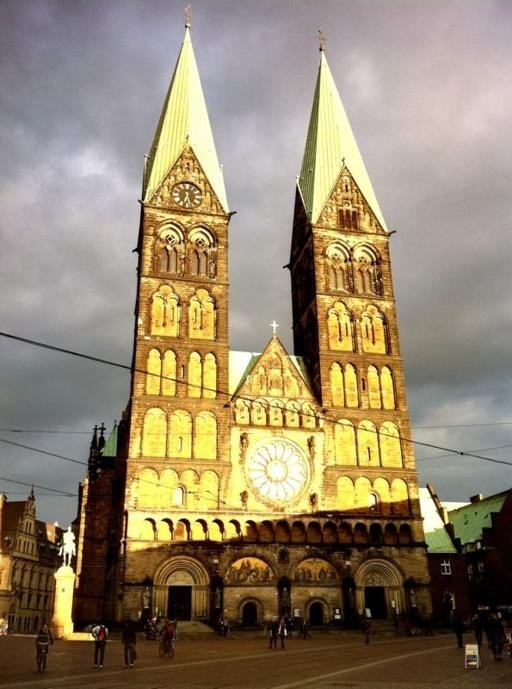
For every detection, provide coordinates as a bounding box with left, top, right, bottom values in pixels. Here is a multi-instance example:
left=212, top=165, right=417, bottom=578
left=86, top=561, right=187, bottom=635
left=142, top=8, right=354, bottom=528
left=133, top=651, right=136, bottom=660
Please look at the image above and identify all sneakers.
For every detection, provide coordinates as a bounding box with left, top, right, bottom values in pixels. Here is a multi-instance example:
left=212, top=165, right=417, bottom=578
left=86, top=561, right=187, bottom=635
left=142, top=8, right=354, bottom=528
left=93, top=664, right=103, bottom=668
left=124, top=664, right=133, bottom=668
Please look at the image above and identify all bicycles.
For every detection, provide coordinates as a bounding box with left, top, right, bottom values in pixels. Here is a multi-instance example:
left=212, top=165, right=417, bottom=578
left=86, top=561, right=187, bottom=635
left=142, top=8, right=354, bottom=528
left=34, top=639, right=50, bottom=674
left=157, top=632, right=174, bottom=658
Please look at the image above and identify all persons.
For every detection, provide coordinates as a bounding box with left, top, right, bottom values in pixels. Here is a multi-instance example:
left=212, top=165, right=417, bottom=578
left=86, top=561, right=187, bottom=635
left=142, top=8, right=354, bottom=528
left=144, top=603, right=512, bottom=661
left=57, top=526, right=76, bottom=557
left=120, top=618, right=136, bottom=668
left=91, top=619, right=108, bottom=668
left=35, top=622, right=53, bottom=673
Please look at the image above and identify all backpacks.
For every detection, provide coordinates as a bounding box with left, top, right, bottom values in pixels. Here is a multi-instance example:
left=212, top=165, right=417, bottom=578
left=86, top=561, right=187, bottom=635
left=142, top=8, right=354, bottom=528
left=96, top=624, right=107, bottom=641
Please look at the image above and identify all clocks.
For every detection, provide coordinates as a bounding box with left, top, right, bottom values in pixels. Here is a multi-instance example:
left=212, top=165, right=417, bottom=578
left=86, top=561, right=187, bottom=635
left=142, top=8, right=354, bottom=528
left=170, top=181, right=203, bottom=209
